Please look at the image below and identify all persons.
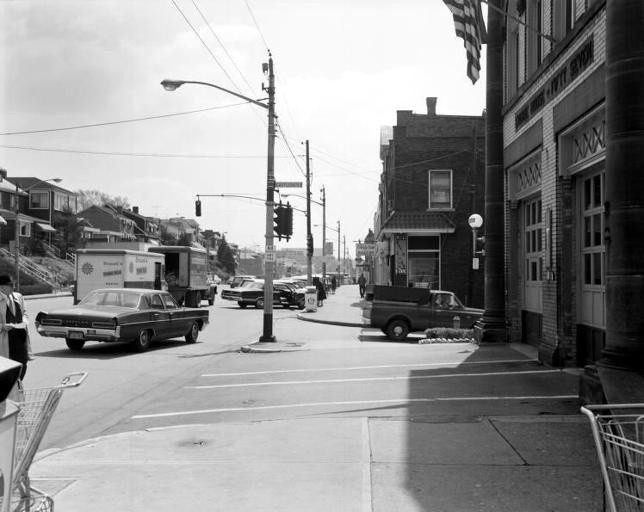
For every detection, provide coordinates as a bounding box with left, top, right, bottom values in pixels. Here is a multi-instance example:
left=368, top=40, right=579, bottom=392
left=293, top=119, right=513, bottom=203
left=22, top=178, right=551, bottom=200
left=358, top=274, right=366, bottom=298
left=0, top=273, right=34, bottom=397
left=331, top=275, right=336, bottom=295
left=313, top=276, right=327, bottom=307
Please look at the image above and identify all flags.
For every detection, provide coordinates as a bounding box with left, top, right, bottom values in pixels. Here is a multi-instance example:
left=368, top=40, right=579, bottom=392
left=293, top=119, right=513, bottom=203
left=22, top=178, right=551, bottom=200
left=446, top=1, right=488, bottom=84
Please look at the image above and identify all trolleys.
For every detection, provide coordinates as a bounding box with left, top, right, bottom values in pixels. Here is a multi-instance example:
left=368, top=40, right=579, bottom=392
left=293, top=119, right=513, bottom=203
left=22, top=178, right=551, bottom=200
left=580, top=402, right=643, bottom=512
left=0, top=368, right=89, bottom=512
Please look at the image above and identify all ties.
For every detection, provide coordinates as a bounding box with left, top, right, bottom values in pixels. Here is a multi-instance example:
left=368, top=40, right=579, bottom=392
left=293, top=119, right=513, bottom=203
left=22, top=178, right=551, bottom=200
left=7, top=294, right=13, bottom=312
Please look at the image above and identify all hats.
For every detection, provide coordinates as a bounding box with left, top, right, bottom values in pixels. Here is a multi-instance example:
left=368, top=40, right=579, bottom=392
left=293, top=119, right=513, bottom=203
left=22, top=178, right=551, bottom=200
left=1, top=273, right=17, bottom=285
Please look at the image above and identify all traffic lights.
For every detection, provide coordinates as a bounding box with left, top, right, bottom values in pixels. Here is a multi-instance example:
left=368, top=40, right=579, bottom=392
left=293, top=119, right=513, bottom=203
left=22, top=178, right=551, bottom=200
left=195, top=200, right=202, bottom=217
left=273, top=206, right=291, bottom=242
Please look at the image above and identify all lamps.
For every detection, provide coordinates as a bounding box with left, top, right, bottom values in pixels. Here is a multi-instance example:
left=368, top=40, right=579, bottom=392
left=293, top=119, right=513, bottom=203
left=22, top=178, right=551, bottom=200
left=469, top=214, right=487, bottom=258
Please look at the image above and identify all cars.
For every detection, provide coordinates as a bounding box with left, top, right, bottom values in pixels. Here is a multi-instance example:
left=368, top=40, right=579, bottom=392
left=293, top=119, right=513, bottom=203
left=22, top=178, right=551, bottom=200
left=34, top=287, right=210, bottom=351
left=221, top=269, right=348, bottom=310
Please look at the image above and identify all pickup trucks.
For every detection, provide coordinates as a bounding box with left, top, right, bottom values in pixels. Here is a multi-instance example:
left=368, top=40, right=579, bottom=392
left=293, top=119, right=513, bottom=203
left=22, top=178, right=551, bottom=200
left=361, top=284, right=485, bottom=343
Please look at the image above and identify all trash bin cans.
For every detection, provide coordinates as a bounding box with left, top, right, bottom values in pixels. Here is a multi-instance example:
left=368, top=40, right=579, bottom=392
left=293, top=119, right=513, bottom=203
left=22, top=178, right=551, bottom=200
left=305, top=286, right=318, bottom=312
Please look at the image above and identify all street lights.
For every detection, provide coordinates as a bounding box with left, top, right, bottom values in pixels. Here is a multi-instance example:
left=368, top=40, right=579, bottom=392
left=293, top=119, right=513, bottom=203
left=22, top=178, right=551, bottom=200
left=280, top=185, right=327, bottom=278
left=160, top=49, right=278, bottom=344
left=14, top=176, right=63, bottom=294
left=312, top=221, right=341, bottom=274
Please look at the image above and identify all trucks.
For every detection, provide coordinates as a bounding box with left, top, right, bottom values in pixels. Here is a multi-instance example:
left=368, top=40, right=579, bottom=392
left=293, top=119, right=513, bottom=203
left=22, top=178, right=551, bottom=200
left=71, top=248, right=168, bottom=305
left=146, top=245, right=218, bottom=308
